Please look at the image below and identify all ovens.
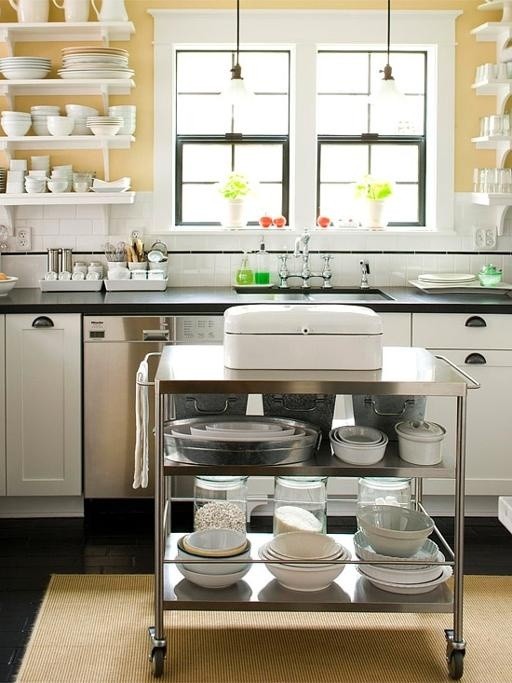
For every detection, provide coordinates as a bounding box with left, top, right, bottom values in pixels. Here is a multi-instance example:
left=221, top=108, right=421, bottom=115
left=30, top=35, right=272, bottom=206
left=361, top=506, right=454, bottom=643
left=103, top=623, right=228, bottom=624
left=79, top=315, right=226, bottom=504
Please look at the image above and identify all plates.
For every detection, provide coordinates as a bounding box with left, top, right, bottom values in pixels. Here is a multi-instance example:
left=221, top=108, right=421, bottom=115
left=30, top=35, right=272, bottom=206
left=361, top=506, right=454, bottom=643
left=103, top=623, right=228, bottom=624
left=0, top=44, right=137, bottom=79
left=233, top=283, right=276, bottom=289
left=409, top=273, right=512, bottom=295
left=89, top=185, right=126, bottom=192
left=158, top=415, right=323, bottom=465
left=36, top=278, right=103, bottom=293
left=101, top=278, right=168, bottom=292
left=350, top=529, right=454, bottom=595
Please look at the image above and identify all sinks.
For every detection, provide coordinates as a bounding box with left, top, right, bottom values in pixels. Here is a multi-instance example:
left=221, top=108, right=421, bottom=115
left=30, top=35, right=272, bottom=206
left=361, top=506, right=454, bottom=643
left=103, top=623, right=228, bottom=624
left=234, top=287, right=309, bottom=302
left=309, top=289, right=395, bottom=304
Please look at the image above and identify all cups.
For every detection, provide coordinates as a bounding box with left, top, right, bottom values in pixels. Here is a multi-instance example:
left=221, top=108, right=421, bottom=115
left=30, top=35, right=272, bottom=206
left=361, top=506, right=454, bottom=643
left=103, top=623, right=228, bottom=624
left=8, top=0, right=129, bottom=22
left=395, top=417, right=447, bottom=466
left=473, top=61, right=512, bottom=193
left=477, top=264, right=503, bottom=288
left=106, top=260, right=170, bottom=282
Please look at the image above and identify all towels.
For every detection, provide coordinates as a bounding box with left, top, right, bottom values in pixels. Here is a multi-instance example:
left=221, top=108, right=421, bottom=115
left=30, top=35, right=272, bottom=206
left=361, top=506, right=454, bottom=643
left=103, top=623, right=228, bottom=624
left=132, top=360, right=149, bottom=490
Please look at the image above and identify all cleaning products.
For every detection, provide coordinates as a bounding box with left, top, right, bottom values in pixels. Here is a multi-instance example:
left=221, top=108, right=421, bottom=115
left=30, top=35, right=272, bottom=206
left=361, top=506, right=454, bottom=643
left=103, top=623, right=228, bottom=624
left=236, top=247, right=254, bottom=285
left=255, top=235, right=270, bottom=284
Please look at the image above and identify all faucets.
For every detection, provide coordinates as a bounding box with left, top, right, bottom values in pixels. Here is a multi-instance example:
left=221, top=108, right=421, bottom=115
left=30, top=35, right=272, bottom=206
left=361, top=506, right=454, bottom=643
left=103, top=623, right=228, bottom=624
left=360, top=259, right=369, bottom=286
left=293, top=227, right=311, bottom=289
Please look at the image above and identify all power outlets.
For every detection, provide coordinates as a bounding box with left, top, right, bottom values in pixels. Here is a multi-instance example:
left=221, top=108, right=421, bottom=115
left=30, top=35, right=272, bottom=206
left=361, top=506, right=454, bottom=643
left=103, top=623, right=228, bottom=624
left=15, top=226, right=33, bottom=252
left=473, top=225, right=498, bottom=250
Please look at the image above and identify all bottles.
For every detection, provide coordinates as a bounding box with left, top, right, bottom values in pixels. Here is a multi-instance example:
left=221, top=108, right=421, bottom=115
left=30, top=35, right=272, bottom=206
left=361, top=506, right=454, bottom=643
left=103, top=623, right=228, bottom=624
left=44, top=246, right=104, bottom=280
left=189, top=475, right=414, bottom=533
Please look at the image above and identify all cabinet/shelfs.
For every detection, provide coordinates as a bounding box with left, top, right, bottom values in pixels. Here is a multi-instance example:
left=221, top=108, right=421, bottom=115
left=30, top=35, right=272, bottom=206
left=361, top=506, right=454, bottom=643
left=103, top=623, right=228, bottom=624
left=412, top=312, right=512, bottom=517
left=0, top=20, right=136, bottom=205
left=471, top=0, right=512, bottom=237
left=0, top=313, right=85, bottom=518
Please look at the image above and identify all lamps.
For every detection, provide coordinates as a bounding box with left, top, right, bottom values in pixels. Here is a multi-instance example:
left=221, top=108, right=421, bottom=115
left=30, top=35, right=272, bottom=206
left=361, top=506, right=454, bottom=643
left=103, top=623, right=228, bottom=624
left=224, top=0, right=249, bottom=103
left=378, top=0, right=399, bottom=103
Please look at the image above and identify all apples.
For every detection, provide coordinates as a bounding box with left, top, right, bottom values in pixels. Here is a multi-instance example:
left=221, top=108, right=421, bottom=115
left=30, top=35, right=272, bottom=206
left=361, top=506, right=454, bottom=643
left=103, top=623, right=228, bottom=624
left=259, top=216, right=286, bottom=227
left=317, top=215, right=331, bottom=227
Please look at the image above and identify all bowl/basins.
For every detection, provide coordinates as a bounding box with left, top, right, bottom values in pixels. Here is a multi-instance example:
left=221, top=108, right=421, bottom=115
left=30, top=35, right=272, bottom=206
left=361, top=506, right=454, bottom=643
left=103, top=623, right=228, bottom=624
left=3, top=153, right=95, bottom=193
left=174, top=529, right=353, bottom=592
left=357, top=502, right=435, bottom=556
left=0, top=273, right=18, bottom=298
left=327, top=425, right=388, bottom=465
left=0, top=102, right=137, bottom=137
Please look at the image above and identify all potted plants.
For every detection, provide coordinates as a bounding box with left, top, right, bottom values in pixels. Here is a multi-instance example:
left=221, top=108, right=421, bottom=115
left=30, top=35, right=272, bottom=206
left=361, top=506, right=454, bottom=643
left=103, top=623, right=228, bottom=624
left=217, top=171, right=251, bottom=227
left=353, top=174, right=393, bottom=227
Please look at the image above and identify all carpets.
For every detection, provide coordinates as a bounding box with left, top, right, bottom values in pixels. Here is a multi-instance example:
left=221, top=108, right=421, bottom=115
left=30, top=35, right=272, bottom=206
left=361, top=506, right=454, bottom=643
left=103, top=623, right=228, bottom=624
left=13, top=574, right=511, bottom=683
